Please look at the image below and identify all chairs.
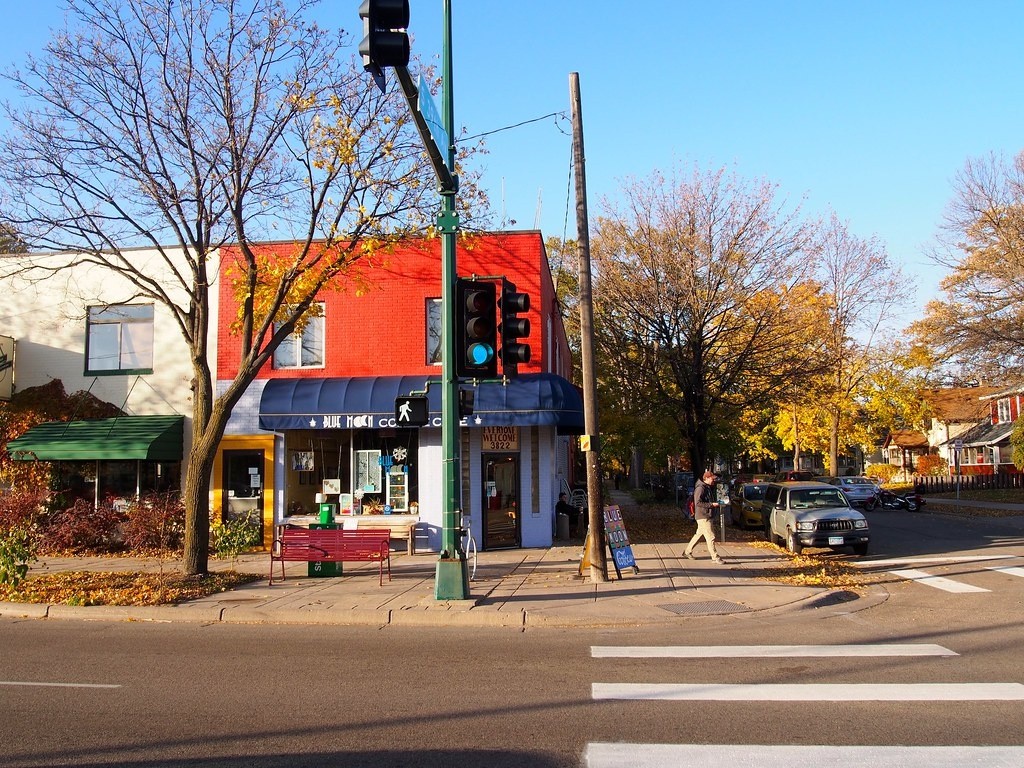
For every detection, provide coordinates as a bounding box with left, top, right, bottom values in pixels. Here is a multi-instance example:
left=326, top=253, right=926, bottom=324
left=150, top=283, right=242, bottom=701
left=814, top=495, right=830, bottom=504
left=790, top=492, right=802, bottom=507
left=560, top=479, right=588, bottom=510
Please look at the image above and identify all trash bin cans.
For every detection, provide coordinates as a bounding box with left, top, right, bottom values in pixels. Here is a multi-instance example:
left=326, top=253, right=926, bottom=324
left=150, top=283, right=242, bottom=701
left=714, top=501, right=733, bottom=527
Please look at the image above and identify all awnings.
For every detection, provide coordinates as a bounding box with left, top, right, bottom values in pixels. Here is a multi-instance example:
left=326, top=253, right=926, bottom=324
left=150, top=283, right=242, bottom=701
left=258, top=373, right=585, bottom=436
left=6, top=414, right=184, bottom=462
left=948, top=422, right=1014, bottom=449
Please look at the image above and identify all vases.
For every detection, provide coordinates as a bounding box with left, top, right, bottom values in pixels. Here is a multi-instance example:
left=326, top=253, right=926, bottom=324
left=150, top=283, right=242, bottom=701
left=410, top=507, right=417, bottom=515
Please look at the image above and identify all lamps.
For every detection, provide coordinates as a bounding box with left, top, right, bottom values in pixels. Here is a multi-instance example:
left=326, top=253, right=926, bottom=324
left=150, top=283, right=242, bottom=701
left=315, top=493, right=326, bottom=515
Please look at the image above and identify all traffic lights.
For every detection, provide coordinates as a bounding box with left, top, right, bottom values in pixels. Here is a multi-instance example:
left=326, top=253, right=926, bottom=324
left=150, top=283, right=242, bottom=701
left=396, top=394, right=435, bottom=430
left=496, top=274, right=534, bottom=382
left=357, top=1, right=415, bottom=93
left=456, top=277, right=500, bottom=380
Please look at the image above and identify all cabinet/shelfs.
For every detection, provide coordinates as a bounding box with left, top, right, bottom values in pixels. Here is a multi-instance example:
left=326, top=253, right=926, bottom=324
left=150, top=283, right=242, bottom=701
left=386, top=472, right=408, bottom=512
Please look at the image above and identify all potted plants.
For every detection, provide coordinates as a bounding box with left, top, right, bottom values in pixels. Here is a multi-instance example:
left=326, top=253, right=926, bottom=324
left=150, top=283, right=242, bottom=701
left=364, top=497, right=384, bottom=515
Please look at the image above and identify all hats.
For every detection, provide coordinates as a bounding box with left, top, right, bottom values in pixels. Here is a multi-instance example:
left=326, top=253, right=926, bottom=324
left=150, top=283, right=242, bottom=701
left=702, top=471, right=717, bottom=479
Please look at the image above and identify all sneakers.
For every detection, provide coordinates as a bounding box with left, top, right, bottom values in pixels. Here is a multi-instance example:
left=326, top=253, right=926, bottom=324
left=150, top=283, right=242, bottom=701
left=711, top=555, right=726, bottom=564
left=681, top=550, right=697, bottom=560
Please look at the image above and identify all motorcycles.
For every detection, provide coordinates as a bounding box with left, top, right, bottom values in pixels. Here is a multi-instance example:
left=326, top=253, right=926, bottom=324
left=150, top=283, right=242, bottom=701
left=864, top=478, right=927, bottom=512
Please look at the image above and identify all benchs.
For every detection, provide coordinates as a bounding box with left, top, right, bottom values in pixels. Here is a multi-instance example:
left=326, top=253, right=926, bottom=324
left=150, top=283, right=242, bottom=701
left=269, top=529, right=391, bottom=585
left=355, top=519, right=417, bottom=555
left=273, top=519, right=345, bottom=554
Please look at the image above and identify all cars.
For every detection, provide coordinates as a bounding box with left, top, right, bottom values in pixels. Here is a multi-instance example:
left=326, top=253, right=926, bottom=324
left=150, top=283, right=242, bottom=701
left=810, top=475, right=837, bottom=487
left=828, top=476, right=877, bottom=505
left=643, top=468, right=820, bottom=510
left=729, top=483, right=774, bottom=531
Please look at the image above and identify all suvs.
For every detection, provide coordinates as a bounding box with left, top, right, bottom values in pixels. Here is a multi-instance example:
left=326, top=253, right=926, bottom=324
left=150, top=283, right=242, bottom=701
left=761, top=481, right=871, bottom=557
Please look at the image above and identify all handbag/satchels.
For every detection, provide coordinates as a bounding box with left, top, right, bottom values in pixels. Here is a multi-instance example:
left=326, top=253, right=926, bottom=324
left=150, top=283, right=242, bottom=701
left=689, top=501, right=695, bottom=516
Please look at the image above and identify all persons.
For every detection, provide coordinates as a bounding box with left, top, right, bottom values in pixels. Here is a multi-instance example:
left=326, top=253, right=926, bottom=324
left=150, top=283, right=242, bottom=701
left=614, top=474, right=620, bottom=490
left=845, top=464, right=856, bottom=476
left=556, top=492, right=589, bottom=534
left=681, top=471, right=726, bottom=564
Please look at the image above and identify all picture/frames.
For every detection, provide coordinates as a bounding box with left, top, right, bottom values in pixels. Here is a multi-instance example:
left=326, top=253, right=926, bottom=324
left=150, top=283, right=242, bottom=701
left=323, top=479, right=340, bottom=494
